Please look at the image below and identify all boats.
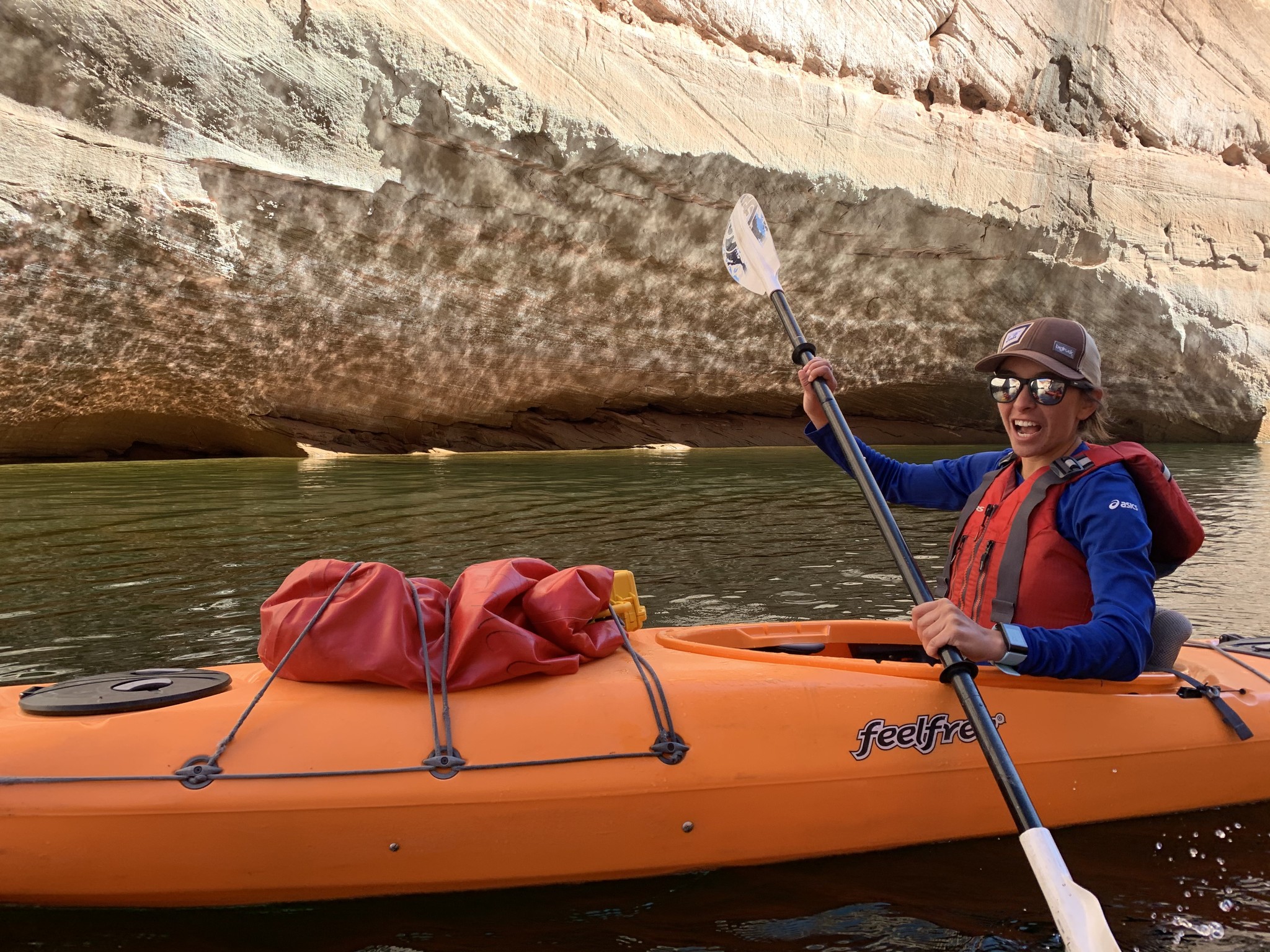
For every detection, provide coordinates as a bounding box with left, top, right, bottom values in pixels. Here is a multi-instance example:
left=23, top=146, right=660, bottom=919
left=0, top=617, right=1268, bottom=907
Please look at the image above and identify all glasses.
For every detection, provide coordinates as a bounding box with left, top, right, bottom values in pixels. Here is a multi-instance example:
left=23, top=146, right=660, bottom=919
left=988, top=375, right=1089, bottom=406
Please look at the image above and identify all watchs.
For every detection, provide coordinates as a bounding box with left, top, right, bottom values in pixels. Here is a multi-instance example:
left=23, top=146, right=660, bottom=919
left=987, top=622, right=1028, bottom=677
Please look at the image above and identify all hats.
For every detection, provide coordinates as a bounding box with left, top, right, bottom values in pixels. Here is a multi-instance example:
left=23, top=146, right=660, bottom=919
left=974, top=317, right=1101, bottom=388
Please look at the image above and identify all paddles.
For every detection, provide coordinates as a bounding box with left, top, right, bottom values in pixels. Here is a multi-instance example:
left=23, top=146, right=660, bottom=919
left=721, top=194, right=1124, bottom=952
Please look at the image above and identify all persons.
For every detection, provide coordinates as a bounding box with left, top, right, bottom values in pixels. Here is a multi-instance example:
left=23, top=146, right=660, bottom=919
left=798, top=317, right=1158, bottom=682
